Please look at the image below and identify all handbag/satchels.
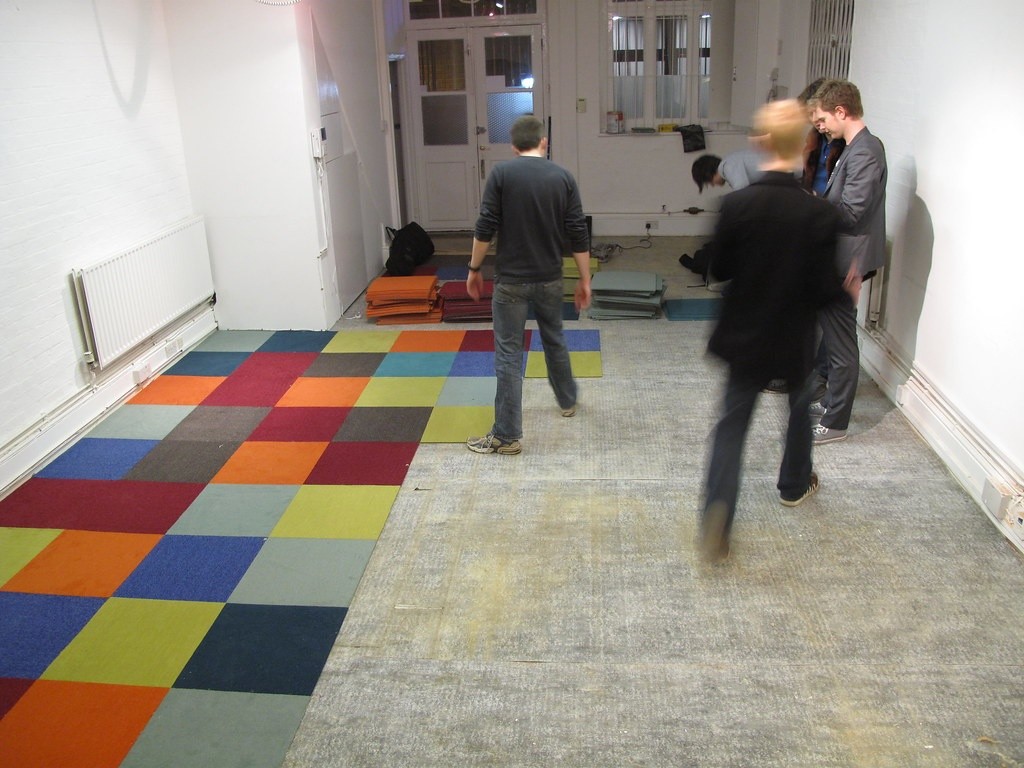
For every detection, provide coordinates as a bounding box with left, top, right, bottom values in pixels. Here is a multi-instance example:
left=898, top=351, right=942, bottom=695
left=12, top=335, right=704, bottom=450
left=694, top=240, right=724, bottom=284
left=385, top=221, right=435, bottom=276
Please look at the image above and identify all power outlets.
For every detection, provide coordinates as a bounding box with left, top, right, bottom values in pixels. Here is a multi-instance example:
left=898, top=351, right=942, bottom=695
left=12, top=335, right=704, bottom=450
left=644, top=221, right=659, bottom=230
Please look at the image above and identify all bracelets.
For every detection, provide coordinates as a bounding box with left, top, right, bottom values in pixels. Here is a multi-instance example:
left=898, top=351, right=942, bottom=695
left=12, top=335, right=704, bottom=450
left=467, top=261, right=481, bottom=272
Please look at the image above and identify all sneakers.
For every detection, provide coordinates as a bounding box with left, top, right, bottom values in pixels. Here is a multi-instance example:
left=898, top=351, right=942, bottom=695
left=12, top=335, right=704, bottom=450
left=808, top=401, right=827, bottom=418
left=466, top=433, right=522, bottom=455
left=696, top=499, right=731, bottom=567
left=559, top=405, right=576, bottom=417
left=780, top=471, right=819, bottom=506
left=810, top=424, right=847, bottom=445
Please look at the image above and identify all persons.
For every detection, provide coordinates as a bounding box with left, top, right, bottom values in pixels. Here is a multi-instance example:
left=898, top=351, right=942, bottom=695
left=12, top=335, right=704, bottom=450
left=697, top=96, right=852, bottom=563
left=691, top=77, right=890, bottom=445
left=466, top=114, right=592, bottom=454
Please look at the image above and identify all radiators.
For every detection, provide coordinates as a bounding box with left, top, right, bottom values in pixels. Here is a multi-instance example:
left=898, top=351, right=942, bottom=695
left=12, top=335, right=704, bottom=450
left=72, top=213, right=214, bottom=369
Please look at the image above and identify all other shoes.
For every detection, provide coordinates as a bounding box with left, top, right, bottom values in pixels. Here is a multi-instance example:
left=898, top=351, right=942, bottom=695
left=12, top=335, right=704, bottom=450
left=761, top=379, right=789, bottom=392
left=802, top=370, right=827, bottom=403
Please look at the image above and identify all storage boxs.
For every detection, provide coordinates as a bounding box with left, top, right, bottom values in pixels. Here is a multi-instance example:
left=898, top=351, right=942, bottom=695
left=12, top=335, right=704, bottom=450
left=658, top=124, right=678, bottom=132
